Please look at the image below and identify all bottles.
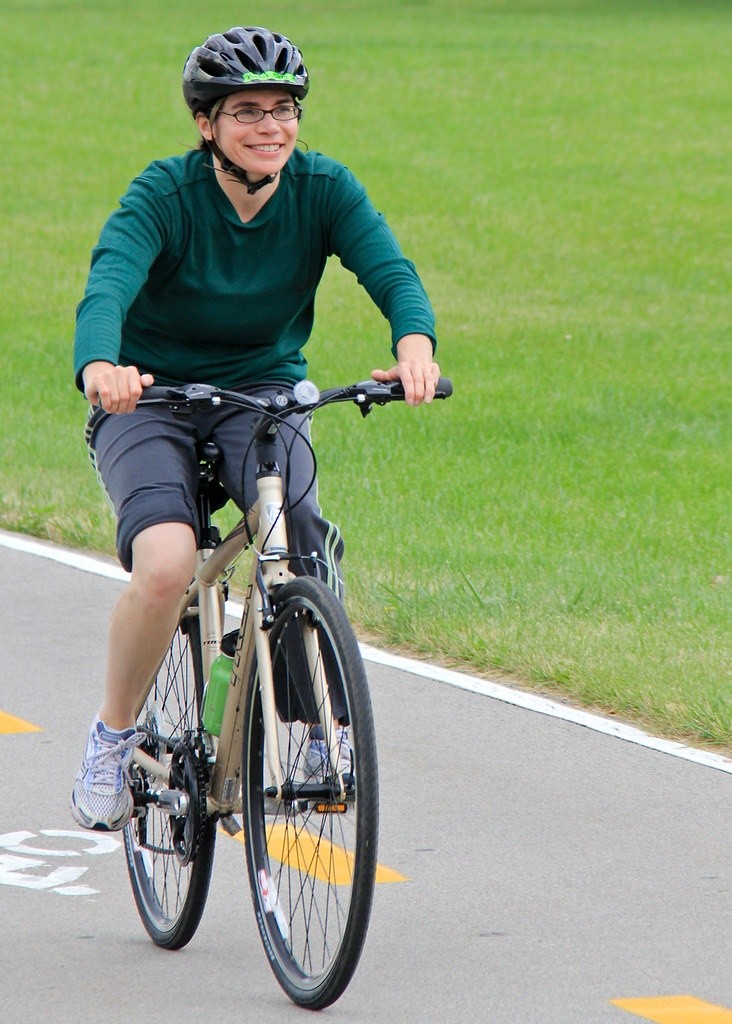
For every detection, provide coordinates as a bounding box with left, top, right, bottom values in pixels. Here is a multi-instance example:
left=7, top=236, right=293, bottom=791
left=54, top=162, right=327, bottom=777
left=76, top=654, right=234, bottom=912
left=204, top=628, right=240, bottom=739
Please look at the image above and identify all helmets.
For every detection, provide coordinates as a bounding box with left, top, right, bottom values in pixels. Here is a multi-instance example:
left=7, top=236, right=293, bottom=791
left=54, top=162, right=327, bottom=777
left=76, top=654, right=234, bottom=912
left=182, top=25, right=311, bottom=105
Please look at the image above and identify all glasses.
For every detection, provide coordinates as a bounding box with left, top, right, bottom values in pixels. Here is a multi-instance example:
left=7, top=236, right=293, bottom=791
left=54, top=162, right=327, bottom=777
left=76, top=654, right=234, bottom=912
left=204, top=99, right=303, bottom=125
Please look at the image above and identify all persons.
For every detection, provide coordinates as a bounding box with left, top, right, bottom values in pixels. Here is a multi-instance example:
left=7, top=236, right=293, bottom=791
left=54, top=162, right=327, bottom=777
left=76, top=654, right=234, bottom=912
left=67, top=25, right=444, bottom=835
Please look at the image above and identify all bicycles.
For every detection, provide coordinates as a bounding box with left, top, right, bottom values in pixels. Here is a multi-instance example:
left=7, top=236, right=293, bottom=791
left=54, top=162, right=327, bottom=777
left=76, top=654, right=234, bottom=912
left=97, top=378, right=454, bottom=1012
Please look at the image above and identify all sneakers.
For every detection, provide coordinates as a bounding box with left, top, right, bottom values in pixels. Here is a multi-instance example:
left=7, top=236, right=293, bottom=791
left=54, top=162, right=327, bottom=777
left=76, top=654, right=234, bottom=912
left=301, top=724, right=355, bottom=790
left=67, top=712, right=140, bottom=832
